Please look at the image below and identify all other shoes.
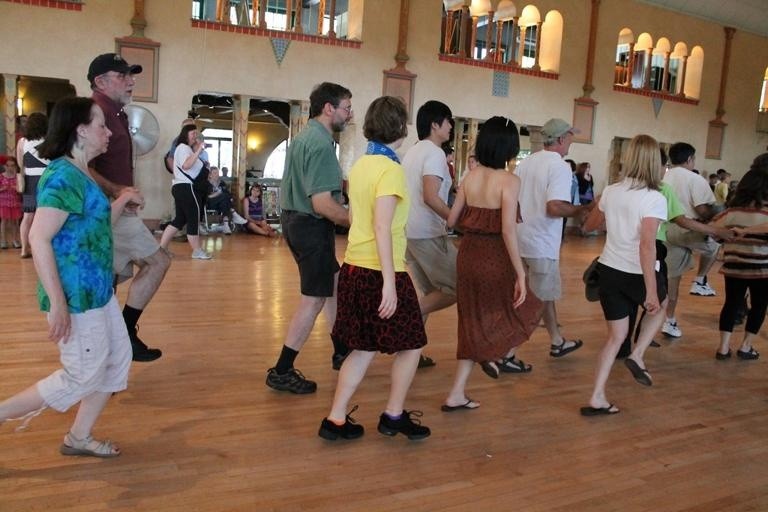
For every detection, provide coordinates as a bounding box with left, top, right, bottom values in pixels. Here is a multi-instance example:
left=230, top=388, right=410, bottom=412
left=634, top=338, right=661, bottom=347
left=614, top=351, right=632, bottom=359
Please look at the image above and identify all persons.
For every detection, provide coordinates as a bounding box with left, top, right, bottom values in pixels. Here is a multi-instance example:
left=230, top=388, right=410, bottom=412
left=267, top=81, right=352, bottom=395
left=1, top=113, right=50, bottom=258
left=493, top=118, right=603, bottom=373
left=88, top=52, right=170, bottom=361
left=1, top=96, right=146, bottom=457
left=165, top=118, right=278, bottom=260
left=560, top=155, right=602, bottom=241
left=440, top=116, right=546, bottom=411
left=444, top=146, right=461, bottom=239
left=579, top=134, right=667, bottom=416
left=400, top=99, right=460, bottom=368
left=461, top=154, right=478, bottom=184
left=661, top=143, right=721, bottom=339
left=336, top=193, right=349, bottom=234
left=691, top=149, right=767, bottom=361
left=318, top=95, right=431, bottom=440
left=615, top=149, right=732, bottom=361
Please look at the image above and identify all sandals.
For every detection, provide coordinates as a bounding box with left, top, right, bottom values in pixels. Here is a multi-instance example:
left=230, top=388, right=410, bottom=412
left=550, top=338, right=583, bottom=357
left=496, top=355, right=532, bottom=372
left=417, top=354, right=437, bottom=368
left=60, top=432, right=121, bottom=457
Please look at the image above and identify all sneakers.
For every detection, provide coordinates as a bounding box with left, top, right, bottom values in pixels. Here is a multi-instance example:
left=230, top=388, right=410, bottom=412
left=333, top=350, right=351, bottom=370
left=0, top=241, right=8, bottom=248
left=266, top=365, right=317, bottom=394
left=661, top=322, right=681, bottom=338
left=13, top=241, right=20, bottom=247
left=161, top=214, right=248, bottom=259
left=319, top=405, right=362, bottom=439
left=128, top=327, right=161, bottom=361
left=689, top=275, right=717, bottom=296
left=378, top=409, right=430, bottom=439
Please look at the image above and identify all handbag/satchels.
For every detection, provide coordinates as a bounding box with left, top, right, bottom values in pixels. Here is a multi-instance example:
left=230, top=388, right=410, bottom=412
left=16, top=173, right=26, bottom=193
left=583, top=256, right=604, bottom=301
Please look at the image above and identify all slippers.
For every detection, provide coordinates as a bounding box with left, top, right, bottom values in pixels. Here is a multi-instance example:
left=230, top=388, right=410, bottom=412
left=716, top=347, right=732, bottom=360
left=479, top=361, right=499, bottom=379
left=580, top=404, right=620, bottom=415
left=442, top=399, right=480, bottom=412
left=624, top=358, right=652, bottom=386
left=737, top=347, right=758, bottom=359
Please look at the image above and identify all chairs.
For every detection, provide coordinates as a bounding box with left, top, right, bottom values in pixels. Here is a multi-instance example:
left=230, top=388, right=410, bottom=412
left=204, top=205, right=236, bottom=232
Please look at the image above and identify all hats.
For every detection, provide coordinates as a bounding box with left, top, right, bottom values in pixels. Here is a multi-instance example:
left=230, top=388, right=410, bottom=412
left=88, top=53, right=143, bottom=79
left=541, top=118, right=581, bottom=145
